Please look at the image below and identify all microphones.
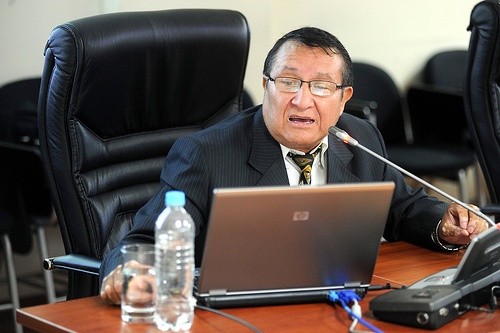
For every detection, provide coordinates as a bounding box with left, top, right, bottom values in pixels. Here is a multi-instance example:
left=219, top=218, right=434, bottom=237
left=328, top=126, right=495, bottom=226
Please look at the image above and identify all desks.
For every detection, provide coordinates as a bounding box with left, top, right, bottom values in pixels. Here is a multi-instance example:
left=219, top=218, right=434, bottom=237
left=16, top=241, right=500, bottom=333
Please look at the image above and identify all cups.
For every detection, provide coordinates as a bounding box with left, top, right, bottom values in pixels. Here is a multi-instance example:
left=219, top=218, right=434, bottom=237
left=119, top=244, right=156, bottom=325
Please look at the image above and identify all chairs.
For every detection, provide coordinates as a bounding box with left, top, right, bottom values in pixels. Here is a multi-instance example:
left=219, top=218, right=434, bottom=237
left=0, top=0, right=500, bottom=333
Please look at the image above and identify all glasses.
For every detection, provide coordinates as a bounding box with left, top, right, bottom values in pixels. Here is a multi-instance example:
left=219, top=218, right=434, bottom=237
left=266, top=74, right=351, bottom=97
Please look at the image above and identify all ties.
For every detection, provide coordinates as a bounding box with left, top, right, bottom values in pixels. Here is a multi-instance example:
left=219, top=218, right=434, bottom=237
left=287, top=144, right=323, bottom=186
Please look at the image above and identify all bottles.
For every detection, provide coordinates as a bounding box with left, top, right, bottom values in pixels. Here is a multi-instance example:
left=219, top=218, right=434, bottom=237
left=154, top=190, right=195, bottom=332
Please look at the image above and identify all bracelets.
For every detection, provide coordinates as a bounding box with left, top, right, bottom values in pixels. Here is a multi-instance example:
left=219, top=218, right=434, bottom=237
left=435, top=219, right=469, bottom=251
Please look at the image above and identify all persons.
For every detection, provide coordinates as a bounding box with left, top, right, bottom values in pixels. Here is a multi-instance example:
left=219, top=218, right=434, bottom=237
left=98, top=26, right=490, bottom=306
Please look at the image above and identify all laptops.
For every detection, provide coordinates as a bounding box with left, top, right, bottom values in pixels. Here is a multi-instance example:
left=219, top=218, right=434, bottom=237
left=191, top=182, right=395, bottom=308
